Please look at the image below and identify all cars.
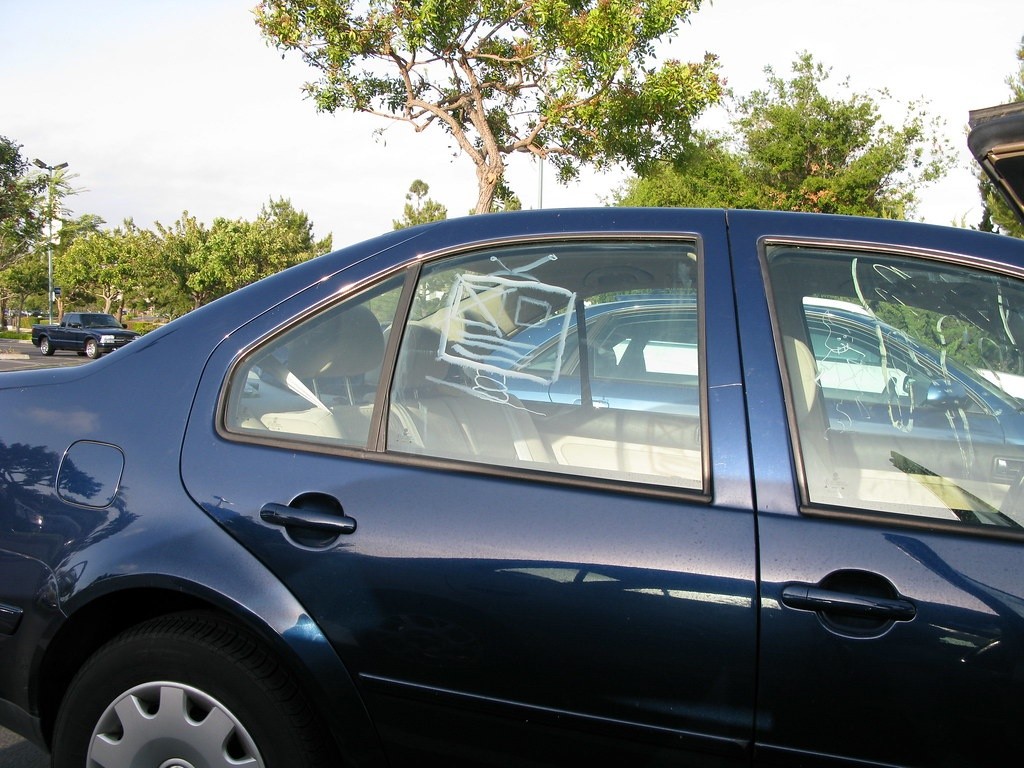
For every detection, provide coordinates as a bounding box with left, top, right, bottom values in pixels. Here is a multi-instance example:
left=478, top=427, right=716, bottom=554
left=482, top=293, right=1024, bottom=444
left=1, top=205, right=1024, bottom=768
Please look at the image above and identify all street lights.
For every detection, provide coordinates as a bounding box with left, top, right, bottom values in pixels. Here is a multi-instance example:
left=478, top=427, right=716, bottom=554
left=32, top=158, right=69, bottom=326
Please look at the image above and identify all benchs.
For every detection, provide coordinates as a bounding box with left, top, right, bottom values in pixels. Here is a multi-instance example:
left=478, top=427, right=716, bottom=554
left=264, top=303, right=553, bottom=462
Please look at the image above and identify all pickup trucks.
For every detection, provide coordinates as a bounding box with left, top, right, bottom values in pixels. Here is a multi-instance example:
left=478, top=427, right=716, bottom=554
left=31, top=313, right=142, bottom=359
